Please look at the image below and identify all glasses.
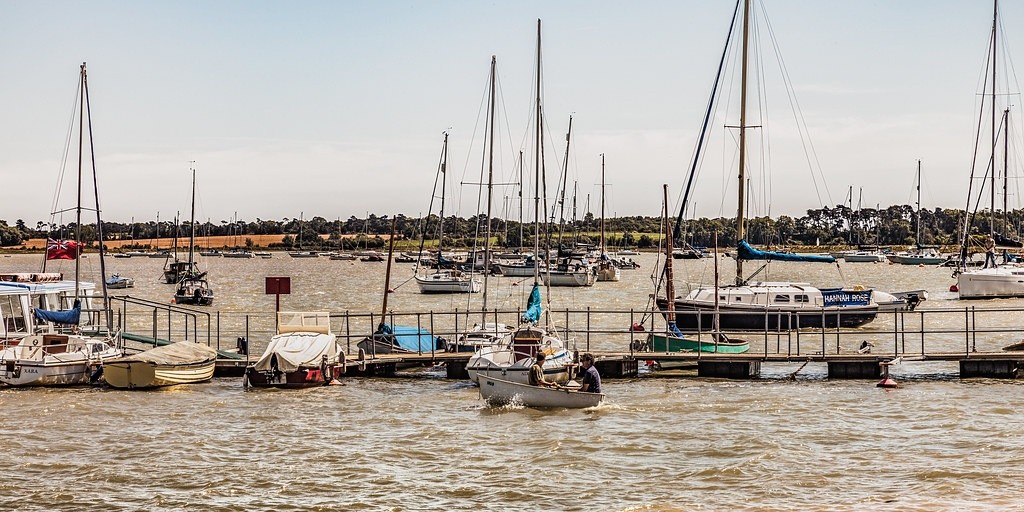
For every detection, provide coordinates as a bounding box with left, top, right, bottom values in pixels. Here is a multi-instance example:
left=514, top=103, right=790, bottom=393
left=582, top=360, right=588, bottom=362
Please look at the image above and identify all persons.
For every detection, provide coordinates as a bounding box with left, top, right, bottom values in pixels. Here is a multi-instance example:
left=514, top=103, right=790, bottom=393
left=528, top=353, right=557, bottom=387
left=578, top=354, right=601, bottom=393
left=984, top=234, right=995, bottom=269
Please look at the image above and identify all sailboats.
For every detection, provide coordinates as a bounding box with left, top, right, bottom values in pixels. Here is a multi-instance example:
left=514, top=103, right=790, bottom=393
left=357, top=19, right=580, bottom=384
left=672, top=110, right=1024, bottom=267
left=954, top=0, right=1024, bottom=297
left=115, top=56, right=638, bottom=305
left=651, top=0, right=928, bottom=329
left=1, top=63, right=121, bottom=389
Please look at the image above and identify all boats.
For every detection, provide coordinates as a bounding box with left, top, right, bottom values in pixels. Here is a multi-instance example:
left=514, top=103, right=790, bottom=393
left=478, top=374, right=605, bottom=408
left=102, top=341, right=218, bottom=390
left=106, top=275, right=135, bottom=289
left=645, top=183, right=752, bottom=354
left=245, top=330, right=347, bottom=389
left=0, top=272, right=96, bottom=321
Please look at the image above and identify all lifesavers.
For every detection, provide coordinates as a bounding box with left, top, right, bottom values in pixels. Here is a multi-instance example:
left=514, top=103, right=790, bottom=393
left=630, top=338, right=648, bottom=351
left=91, top=366, right=103, bottom=383
left=242, top=374, right=248, bottom=387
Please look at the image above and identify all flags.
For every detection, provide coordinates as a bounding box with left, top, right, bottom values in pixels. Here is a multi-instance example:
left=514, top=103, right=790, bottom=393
left=47, top=238, right=83, bottom=260
